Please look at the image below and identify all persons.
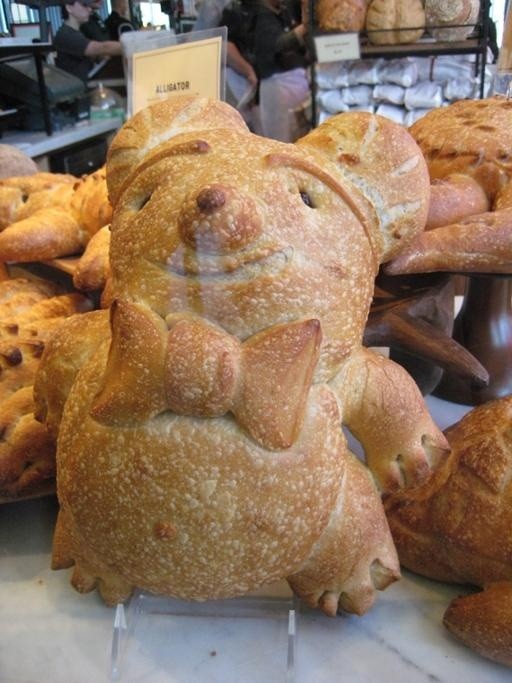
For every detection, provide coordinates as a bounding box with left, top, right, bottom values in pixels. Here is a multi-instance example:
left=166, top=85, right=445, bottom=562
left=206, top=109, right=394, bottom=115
left=486, top=0, right=498, bottom=64
left=52, top=0, right=315, bottom=144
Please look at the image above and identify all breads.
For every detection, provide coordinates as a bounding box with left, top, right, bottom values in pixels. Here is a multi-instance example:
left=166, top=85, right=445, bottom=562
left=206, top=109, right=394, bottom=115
left=29, top=96, right=406, bottom=621
left=0, top=261, right=97, bottom=507
left=313, top=1, right=482, bottom=47
left=0, top=165, right=114, bottom=293
left=379, top=394, right=511, bottom=668
left=380, top=95, right=512, bottom=276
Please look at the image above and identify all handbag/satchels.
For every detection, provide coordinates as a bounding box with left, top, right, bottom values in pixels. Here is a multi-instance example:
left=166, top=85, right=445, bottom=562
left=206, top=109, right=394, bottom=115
left=273, top=51, right=310, bottom=70
